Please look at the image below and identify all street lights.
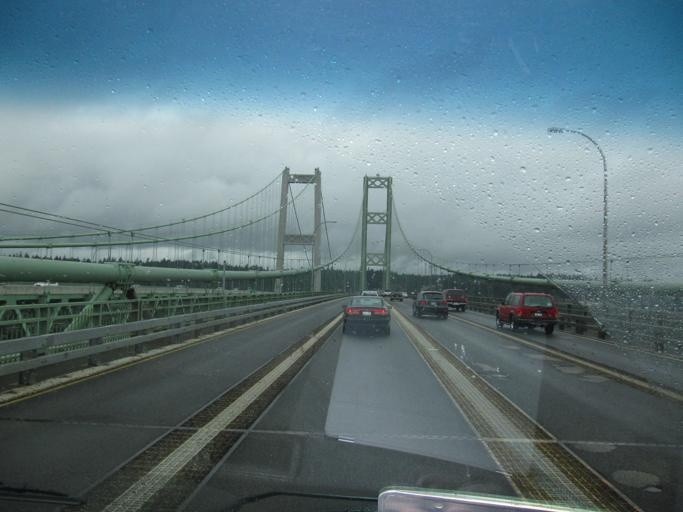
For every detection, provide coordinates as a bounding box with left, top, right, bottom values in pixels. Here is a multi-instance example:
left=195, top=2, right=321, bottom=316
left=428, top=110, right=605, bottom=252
left=547, top=127, right=609, bottom=281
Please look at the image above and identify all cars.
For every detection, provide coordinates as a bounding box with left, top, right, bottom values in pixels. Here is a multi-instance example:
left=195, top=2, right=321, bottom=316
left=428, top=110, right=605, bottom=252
left=495, top=291, right=560, bottom=333
left=391, top=292, right=403, bottom=302
left=341, top=295, right=393, bottom=337
left=411, top=290, right=449, bottom=320
left=442, top=288, right=467, bottom=313
